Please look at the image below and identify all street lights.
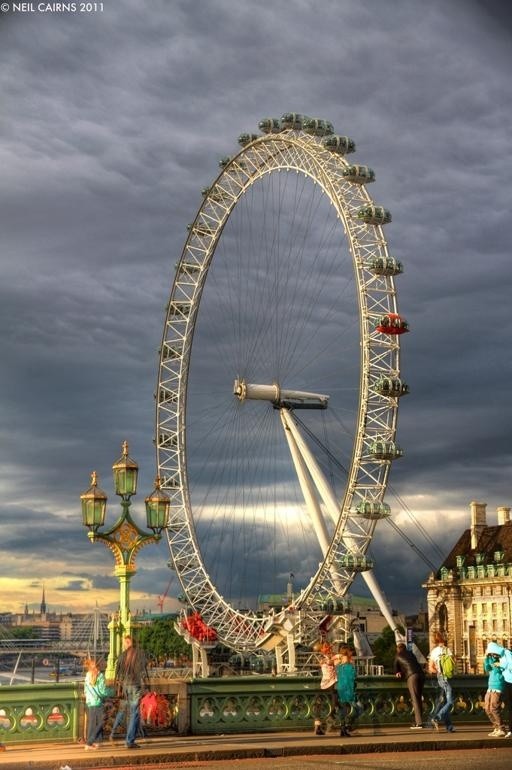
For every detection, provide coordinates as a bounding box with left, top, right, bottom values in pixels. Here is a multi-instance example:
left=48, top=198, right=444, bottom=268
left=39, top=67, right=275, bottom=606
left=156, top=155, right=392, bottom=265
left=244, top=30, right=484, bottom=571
left=78, top=439, right=171, bottom=682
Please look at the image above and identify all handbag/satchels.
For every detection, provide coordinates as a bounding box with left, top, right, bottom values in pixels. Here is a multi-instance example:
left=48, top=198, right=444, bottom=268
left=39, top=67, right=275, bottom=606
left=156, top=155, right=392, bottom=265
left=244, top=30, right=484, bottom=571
left=140, top=692, right=172, bottom=732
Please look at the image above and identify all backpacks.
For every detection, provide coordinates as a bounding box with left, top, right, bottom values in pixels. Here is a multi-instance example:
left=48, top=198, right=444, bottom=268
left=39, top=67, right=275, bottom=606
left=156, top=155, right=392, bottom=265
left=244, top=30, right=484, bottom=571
left=439, top=646, right=455, bottom=679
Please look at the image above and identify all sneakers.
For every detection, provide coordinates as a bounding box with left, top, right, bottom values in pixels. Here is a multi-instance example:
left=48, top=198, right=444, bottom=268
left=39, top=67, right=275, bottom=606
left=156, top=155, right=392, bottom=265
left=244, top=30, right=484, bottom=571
left=409, top=723, right=423, bottom=730
left=84, top=744, right=97, bottom=750
left=315, top=723, right=325, bottom=735
left=340, top=725, right=351, bottom=738
left=430, top=715, right=456, bottom=734
left=488, top=728, right=512, bottom=739
left=127, top=743, right=141, bottom=749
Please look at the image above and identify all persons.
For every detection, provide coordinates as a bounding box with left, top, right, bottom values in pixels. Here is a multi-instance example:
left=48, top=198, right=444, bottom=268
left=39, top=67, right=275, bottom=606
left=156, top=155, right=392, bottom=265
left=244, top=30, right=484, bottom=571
left=428, top=630, right=460, bottom=734
left=487, top=642, right=512, bottom=738
left=393, top=642, right=424, bottom=731
left=110, top=636, right=155, bottom=751
left=483, top=648, right=508, bottom=738
left=312, top=641, right=364, bottom=737
left=83, top=656, right=120, bottom=751
